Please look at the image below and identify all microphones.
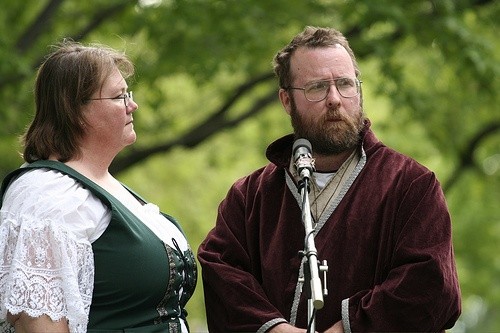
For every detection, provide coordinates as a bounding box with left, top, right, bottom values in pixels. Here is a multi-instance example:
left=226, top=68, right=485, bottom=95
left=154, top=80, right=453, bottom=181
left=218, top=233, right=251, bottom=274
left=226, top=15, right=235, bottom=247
left=293, top=138, right=313, bottom=181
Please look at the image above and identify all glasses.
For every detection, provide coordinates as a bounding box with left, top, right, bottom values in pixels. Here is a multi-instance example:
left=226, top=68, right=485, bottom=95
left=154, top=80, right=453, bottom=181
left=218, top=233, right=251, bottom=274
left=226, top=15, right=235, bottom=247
left=82, top=90, right=133, bottom=106
left=285, top=76, right=363, bottom=102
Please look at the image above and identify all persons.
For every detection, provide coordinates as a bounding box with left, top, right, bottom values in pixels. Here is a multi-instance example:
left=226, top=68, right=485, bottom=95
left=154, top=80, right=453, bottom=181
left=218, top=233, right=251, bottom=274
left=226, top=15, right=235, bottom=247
left=0, top=42, right=198, bottom=333
left=196, top=25, right=462, bottom=333
